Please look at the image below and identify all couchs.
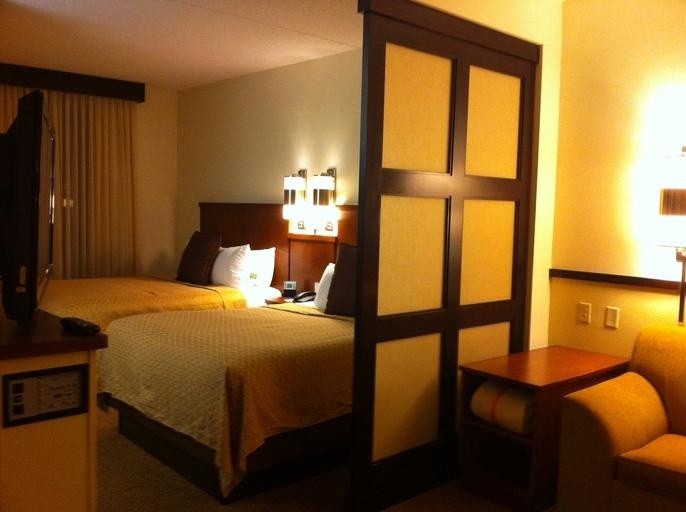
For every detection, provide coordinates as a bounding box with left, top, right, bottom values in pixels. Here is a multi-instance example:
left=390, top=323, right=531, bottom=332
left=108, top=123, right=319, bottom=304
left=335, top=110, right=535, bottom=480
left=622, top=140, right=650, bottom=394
left=562, top=327, right=686, bottom=512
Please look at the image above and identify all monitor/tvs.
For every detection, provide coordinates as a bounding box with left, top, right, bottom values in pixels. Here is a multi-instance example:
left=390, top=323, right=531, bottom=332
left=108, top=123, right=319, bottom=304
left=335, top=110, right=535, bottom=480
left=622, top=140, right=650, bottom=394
left=0, top=88, right=56, bottom=326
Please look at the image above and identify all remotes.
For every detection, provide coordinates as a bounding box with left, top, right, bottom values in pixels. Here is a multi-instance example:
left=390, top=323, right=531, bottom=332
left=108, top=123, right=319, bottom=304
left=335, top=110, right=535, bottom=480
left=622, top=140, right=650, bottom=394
left=61, top=317, right=100, bottom=334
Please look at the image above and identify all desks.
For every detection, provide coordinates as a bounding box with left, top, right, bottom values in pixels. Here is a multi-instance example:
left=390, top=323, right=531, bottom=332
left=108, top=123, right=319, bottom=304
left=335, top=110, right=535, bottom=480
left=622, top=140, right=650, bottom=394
left=0, top=310, right=110, bottom=512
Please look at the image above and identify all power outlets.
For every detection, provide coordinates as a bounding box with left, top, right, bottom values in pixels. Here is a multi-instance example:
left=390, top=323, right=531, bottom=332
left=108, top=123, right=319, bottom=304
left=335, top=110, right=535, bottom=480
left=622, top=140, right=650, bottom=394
left=575, top=302, right=592, bottom=324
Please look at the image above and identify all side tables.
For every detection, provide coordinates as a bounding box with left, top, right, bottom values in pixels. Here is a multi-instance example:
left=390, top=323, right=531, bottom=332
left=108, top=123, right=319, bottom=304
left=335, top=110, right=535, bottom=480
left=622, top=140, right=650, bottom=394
left=456, top=344, right=628, bottom=505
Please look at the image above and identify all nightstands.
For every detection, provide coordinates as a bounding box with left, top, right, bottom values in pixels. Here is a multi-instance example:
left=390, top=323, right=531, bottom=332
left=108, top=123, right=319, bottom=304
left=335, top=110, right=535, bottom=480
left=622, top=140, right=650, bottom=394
left=264, top=293, right=312, bottom=306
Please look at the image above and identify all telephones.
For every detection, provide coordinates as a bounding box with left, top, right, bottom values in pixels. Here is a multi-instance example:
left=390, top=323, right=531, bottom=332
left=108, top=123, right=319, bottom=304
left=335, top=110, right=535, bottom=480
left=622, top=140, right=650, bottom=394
left=294, top=291, right=316, bottom=302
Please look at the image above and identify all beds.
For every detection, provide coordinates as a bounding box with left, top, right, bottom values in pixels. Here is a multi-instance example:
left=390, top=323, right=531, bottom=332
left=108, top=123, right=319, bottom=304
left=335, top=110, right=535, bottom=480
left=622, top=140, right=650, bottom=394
left=96, top=202, right=353, bottom=496
left=37, top=198, right=290, bottom=331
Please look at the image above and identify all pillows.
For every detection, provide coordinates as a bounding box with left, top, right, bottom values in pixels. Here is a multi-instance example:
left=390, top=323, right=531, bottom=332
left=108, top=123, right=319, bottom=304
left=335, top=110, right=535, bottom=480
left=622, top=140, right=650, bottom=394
left=174, top=230, right=221, bottom=283
left=320, top=241, right=358, bottom=315
left=249, top=247, right=274, bottom=291
left=313, top=261, right=334, bottom=310
left=210, top=243, right=250, bottom=286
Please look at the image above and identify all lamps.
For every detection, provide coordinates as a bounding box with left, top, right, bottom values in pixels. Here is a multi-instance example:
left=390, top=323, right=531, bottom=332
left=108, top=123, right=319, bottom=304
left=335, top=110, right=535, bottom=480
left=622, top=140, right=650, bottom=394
left=309, top=170, right=340, bottom=234
left=648, top=133, right=686, bottom=334
left=281, top=171, right=309, bottom=231
